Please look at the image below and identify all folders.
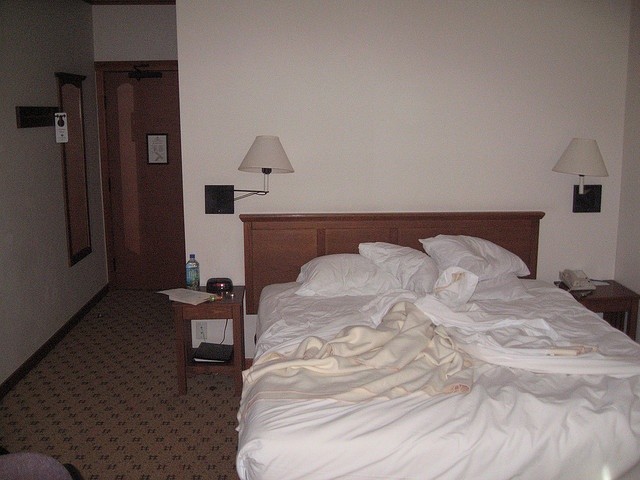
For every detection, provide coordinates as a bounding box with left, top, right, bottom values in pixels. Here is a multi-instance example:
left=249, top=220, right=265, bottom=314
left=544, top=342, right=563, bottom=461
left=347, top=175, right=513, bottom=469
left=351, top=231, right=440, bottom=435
left=192, top=342, right=233, bottom=363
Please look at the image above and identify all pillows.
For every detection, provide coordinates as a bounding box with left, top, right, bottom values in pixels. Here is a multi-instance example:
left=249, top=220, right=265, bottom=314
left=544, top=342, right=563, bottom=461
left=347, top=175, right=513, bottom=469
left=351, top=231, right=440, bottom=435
left=464, top=273, right=535, bottom=304
left=416, top=233, right=532, bottom=282
left=294, top=254, right=396, bottom=298
left=357, top=239, right=438, bottom=295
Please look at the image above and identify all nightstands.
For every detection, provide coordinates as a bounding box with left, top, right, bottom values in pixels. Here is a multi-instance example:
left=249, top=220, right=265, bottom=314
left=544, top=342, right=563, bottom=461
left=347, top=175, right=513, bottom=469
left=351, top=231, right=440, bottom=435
left=551, top=278, right=639, bottom=342
left=170, top=285, right=246, bottom=397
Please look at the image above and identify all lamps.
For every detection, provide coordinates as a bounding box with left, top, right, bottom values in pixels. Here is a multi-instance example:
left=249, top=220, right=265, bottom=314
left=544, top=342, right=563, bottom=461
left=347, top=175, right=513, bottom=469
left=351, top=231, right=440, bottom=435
left=550, top=137, right=609, bottom=197
left=236, top=135, right=295, bottom=193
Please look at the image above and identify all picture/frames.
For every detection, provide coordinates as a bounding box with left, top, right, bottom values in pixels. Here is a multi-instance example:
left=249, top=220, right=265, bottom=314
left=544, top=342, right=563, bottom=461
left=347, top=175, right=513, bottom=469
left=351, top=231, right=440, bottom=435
left=144, top=132, right=170, bottom=167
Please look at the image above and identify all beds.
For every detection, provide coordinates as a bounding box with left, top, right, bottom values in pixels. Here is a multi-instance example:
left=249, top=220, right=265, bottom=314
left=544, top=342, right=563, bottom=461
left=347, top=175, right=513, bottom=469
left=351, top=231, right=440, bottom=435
left=238, top=211, right=640, bottom=480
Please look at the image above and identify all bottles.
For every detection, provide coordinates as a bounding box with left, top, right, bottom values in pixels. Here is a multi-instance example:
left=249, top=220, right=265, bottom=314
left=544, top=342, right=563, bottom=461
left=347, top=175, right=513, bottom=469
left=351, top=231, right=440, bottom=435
left=186, top=254, right=200, bottom=290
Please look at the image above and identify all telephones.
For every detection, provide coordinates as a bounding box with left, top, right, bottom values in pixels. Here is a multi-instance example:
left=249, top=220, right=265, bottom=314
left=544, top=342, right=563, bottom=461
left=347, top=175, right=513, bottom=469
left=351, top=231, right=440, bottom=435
left=556, top=269, right=597, bottom=291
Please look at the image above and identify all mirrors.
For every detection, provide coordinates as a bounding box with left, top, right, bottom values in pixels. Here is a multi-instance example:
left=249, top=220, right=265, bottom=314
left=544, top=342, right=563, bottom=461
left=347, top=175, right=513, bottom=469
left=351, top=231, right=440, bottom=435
left=54, top=70, right=94, bottom=268
